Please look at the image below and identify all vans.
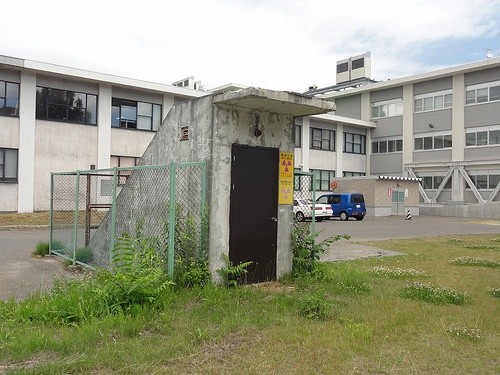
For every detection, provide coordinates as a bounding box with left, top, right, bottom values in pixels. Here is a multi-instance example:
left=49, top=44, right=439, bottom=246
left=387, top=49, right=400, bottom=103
left=316, top=193, right=367, bottom=221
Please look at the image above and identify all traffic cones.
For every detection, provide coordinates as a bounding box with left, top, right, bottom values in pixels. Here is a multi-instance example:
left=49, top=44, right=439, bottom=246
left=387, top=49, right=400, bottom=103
left=404, top=210, right=413, bottom=220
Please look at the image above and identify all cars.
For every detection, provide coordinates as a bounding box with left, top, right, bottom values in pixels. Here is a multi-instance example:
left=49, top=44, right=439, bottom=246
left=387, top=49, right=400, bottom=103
left=293, top=198, right=333, bottom=222
